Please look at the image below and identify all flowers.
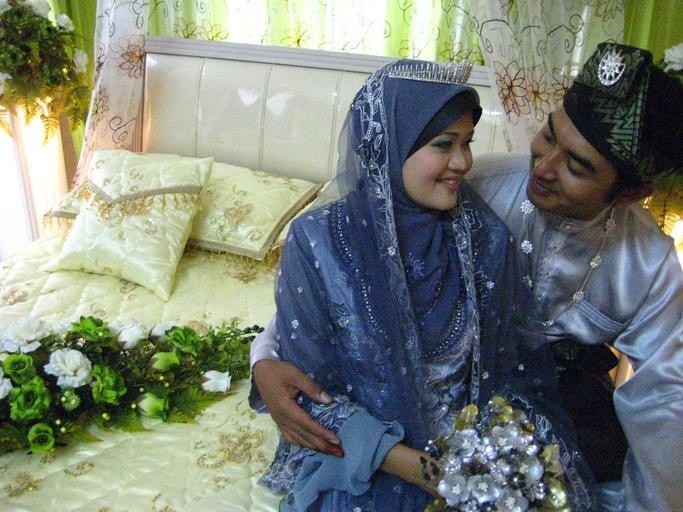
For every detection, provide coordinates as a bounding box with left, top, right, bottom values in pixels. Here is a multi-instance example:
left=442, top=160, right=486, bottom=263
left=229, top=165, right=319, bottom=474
left=0, top=1, right=94, bottom=142
left=1, top=315, right=266, bottom=457
left=417, top=390, right=572, bottom=512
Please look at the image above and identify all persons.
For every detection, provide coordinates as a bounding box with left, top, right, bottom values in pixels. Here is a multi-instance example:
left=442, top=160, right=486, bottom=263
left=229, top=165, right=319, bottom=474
left=261, top=57, right=599, bottom=511
left=247, top=43, right=683, bottom=512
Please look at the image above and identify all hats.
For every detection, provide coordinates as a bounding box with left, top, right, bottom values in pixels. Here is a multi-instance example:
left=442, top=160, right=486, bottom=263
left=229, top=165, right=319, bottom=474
left=562, top=41, right=683, bottom=184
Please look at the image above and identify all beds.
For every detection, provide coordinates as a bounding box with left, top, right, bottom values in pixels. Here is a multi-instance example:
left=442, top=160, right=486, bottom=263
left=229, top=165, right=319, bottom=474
left=0, top=36, right=577, bottom=510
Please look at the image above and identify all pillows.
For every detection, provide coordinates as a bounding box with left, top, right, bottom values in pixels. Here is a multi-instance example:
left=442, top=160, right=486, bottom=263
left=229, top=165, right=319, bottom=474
left=47, top=145, right=321, bottom=260
left=269, top=176, right=360, bottom=252
left=43, top=150, right=215, bottom=302
